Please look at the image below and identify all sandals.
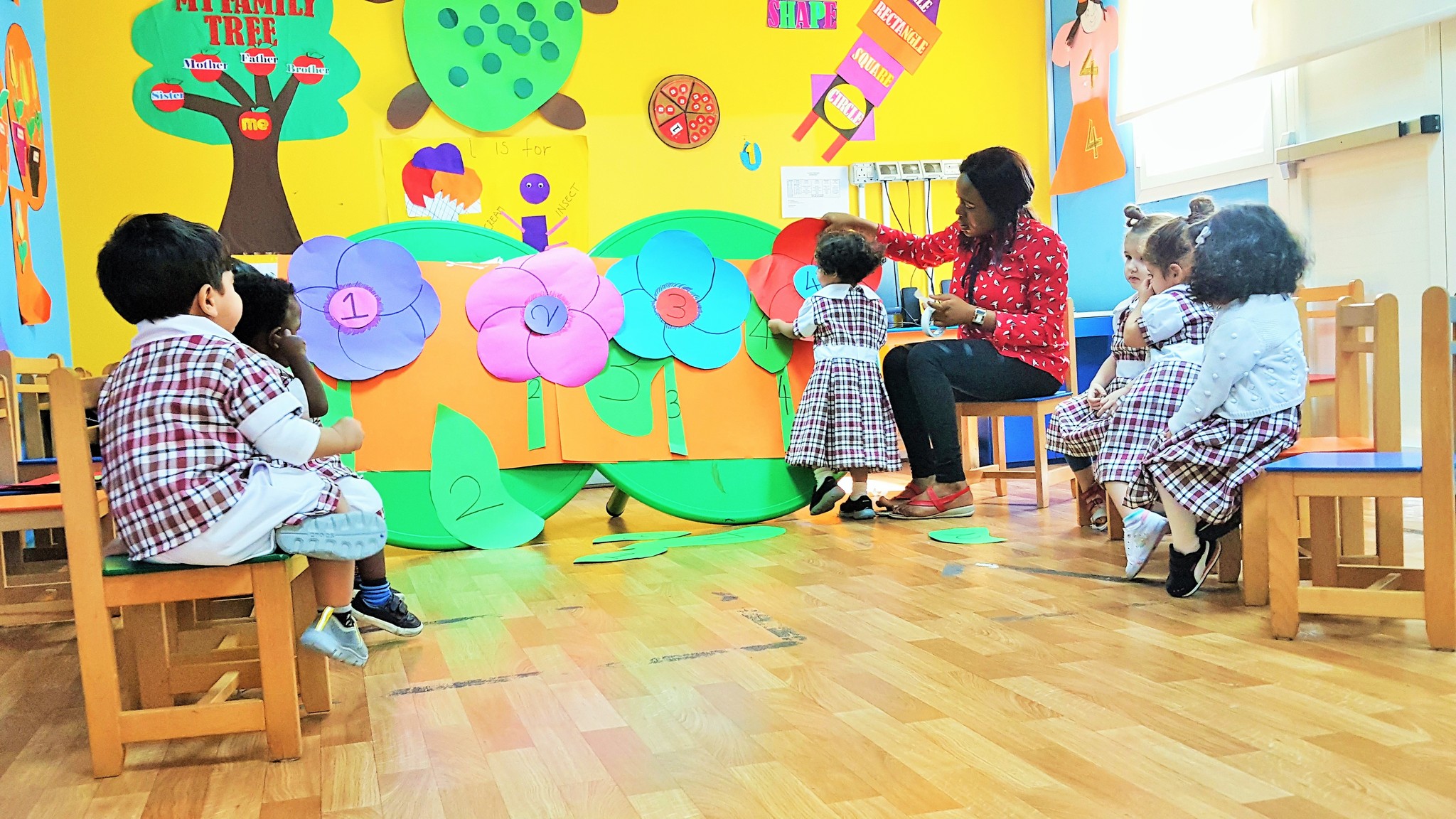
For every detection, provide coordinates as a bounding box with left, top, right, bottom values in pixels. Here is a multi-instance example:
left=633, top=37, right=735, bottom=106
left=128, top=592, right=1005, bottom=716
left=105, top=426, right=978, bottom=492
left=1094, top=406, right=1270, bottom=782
left=875, top=483, right=976, bottom=520
left=1080, top=481, right=1108, bottom=531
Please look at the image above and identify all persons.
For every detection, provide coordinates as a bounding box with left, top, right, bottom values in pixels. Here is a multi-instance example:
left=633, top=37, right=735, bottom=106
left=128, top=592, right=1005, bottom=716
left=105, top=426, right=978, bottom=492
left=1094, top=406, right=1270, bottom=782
left=1090, top=194, right=1220, bottom=582
left=814, top=146, right=1070, bottom=518
left=768, top=228, right=903, bottom=520
left=94, top=210, right=388, bottom=666
left=233, top=274, right=424, bottom=638
left=1121, top=203, right=1310, bottom=599
left=1045, top=205, right=1179, bottom=532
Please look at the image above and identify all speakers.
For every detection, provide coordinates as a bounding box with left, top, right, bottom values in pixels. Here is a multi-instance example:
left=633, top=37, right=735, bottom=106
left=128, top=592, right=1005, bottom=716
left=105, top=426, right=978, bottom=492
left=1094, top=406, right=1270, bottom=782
left=940, top=278, right=952, bottom=294
left=903, top=287, right=922, bottom=326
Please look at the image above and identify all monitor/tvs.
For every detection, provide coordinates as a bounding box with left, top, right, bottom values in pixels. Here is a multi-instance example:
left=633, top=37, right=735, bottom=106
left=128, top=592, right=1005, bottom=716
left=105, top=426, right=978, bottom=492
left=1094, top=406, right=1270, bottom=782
left=874, top=255, right=900, bottom=313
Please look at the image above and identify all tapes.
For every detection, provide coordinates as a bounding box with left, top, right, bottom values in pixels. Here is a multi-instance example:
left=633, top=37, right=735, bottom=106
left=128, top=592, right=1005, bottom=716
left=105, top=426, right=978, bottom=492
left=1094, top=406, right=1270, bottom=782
left=914, top=290, right=946, bottom=338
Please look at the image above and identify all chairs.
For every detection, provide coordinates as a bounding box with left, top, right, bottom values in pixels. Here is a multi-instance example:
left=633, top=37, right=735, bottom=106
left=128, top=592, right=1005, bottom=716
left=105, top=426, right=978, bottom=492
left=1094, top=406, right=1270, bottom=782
left=1264, top=282, right=1456, bottom=653
left=1242, top=293, right=1402, bottom=606
left=954, top=295, right=1076, bottom=511
left=0, top=349, right=334, bottom=779
left=1211, top=279, right=1366, bottom=584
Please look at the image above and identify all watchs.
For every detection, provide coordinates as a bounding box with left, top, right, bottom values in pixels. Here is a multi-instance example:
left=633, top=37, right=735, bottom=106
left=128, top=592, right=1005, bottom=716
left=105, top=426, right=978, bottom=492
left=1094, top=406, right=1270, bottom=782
left=971, top=306, right=988, bottom=327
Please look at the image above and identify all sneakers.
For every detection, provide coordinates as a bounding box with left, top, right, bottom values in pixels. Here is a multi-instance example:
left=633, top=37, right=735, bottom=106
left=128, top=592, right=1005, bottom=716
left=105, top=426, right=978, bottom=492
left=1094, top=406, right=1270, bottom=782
left=1195, top=508, right=1242, bottom=540
left=350, top=590, right=424, bottom=637
left=301, top=605, right=370, bottom=668
left=276, top=510, right=388, bottom=562
left=809, top=476, right=846, bottom=516
left=352, top=579, right=405, bottom=602
left=1123, top=511, right=1168, bottom=581
left=1165, top=538, right=1222, bottom=598
left=838, top=495, right=876, bottom=520
